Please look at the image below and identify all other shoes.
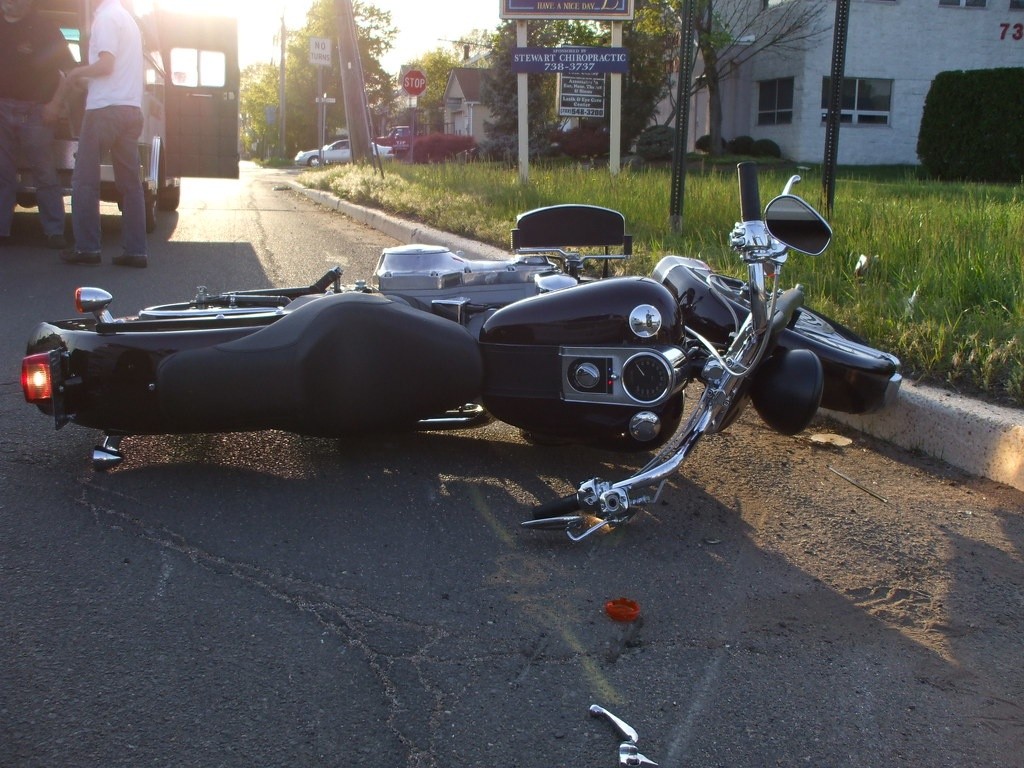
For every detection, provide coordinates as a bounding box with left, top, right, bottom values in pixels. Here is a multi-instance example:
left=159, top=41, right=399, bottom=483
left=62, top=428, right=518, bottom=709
left=43, top=233, right=64, bottom=248
left=1, top=236, right=12, bottom=245
left=111, top=254, right=147, bottom=268
left=61, top=249, right=101, bottom=265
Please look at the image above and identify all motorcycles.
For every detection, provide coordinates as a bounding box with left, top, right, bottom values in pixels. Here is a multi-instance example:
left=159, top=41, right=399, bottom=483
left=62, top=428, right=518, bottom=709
left=19, top=159, right=903, bottom=541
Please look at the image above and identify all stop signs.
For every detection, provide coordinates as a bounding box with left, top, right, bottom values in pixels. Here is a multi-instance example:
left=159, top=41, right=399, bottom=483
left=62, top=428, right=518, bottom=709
left=403, top=71, right=426, bottom=97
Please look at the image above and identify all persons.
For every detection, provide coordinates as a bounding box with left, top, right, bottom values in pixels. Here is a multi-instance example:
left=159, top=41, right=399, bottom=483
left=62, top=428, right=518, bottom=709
left=62, top=0, right=149, bottom=268
left=0, top=0, right=78, bottom=249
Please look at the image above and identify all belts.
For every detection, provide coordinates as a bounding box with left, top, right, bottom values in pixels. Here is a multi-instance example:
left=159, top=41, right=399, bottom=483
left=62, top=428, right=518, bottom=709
left=1, top=103, right=36, bottom=116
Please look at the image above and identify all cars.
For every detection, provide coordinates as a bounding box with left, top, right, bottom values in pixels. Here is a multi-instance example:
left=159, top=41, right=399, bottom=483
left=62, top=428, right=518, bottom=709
left=294, top=137, right=396, bottom=168
left=375, top=125, right=420, bottom=152
left=454, top=134, right=565, bottom=162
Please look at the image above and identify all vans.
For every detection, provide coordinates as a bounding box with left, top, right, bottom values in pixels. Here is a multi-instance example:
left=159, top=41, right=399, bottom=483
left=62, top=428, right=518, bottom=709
left=0, top=0, right=242, bottom=235
